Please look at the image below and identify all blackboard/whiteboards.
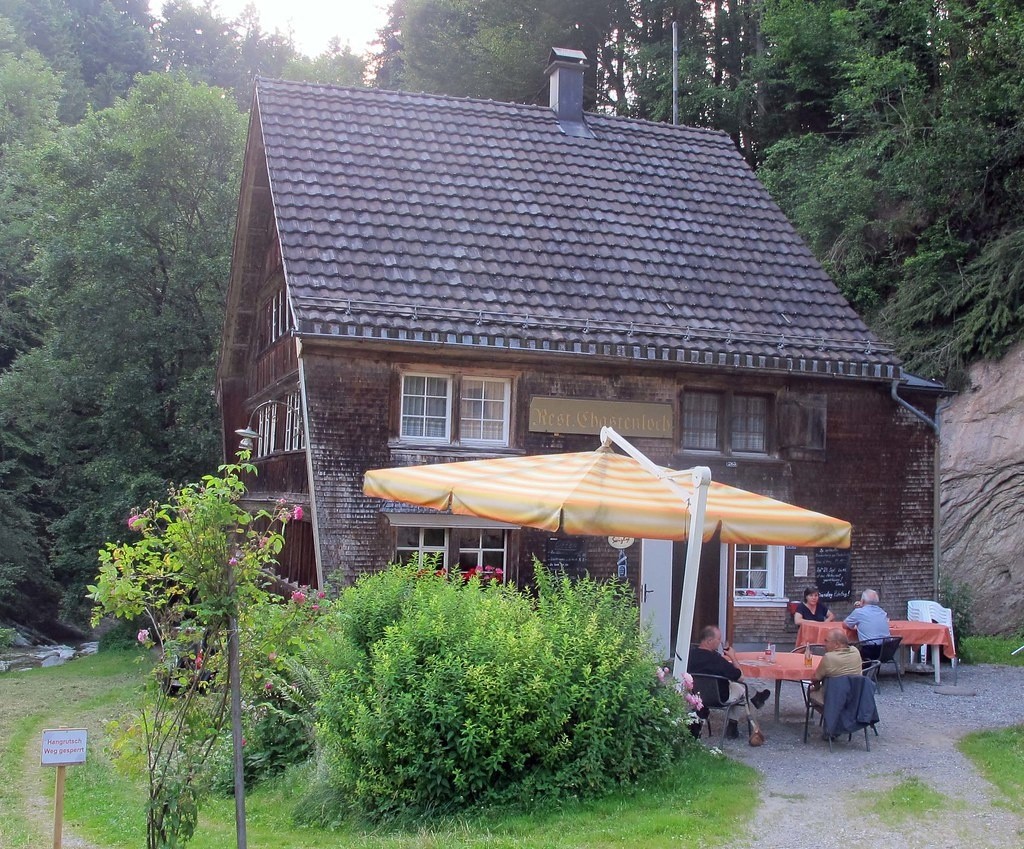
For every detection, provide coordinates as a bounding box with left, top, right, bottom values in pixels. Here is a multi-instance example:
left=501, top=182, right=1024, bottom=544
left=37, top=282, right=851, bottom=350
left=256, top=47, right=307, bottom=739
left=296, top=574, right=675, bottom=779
left=815, top=545, right=854, bottom=604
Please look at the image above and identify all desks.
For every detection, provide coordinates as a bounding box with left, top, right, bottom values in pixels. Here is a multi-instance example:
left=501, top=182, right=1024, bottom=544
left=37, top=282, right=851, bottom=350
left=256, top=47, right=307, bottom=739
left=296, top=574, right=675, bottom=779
left=720, top=651, right=824, bottom=721
left=796, top=620, right=955, bottom=683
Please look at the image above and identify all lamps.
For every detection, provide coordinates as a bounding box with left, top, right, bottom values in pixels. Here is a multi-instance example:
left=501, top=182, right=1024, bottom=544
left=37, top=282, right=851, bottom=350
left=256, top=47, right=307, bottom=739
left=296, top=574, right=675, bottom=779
left=235, top=399, right=304, bottom=450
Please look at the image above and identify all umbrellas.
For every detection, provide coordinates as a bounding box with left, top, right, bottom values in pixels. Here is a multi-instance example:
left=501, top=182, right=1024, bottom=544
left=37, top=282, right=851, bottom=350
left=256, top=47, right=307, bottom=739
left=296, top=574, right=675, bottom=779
left=360, top=450, right=851, bottom=691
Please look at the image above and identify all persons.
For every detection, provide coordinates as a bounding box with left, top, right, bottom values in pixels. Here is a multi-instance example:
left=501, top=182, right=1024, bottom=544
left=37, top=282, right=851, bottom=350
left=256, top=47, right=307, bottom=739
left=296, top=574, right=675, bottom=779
left=842, top=589, right=890, bottom=645
left=794, top=587, right=835, bottom=627
left=807, top=628, right=862, bottom=741
left=688, top=626, right=770, bottom=739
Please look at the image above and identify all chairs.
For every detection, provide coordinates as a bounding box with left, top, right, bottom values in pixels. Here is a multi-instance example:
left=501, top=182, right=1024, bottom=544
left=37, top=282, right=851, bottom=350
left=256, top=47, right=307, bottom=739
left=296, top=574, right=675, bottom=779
left=690, top=673, right=752, bottom=750
left=853, top=636, right=904, bottom=693
left=790, top=643, right=826, bottom=706
left=907, top=600, right=955, bottom=667
left=803, top=659, right=881, bottom=751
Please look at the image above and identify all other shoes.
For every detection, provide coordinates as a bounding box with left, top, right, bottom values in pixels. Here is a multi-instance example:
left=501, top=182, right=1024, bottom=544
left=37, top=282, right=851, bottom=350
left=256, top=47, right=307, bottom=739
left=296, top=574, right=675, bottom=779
left=726, top=719, right=739, bottom=738
left=750, top=689, right=771, bottom=709
left=823, top=735, right=836, bottom=740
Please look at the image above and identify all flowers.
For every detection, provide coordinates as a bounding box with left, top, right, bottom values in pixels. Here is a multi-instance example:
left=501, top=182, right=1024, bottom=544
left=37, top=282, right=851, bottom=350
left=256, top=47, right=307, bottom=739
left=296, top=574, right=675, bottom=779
left=656, top=666, right=720, bottom=759
left=418, top=561, right=503, bottom=589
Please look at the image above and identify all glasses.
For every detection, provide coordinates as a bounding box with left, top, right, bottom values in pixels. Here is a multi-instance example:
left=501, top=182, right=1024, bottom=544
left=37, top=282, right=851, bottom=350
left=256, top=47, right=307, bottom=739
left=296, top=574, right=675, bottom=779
left=825, top=639, right=837, bottom=642
left=808, top=586, right=819, bottom=589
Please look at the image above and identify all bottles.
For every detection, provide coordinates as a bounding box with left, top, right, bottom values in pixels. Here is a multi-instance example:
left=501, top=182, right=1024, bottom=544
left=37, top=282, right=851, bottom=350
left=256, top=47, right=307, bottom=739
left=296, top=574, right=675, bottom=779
left=765, top=642, right=771, bottom=661
left=805, top=641, right=812, bottom=667
left=770, top=645, right=776, bottom=662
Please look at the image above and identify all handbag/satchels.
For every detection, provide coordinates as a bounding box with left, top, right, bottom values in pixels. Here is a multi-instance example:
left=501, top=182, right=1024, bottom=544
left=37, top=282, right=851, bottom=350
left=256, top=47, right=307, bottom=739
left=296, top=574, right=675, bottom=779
left=748, top=710, right=764, bottom=746
